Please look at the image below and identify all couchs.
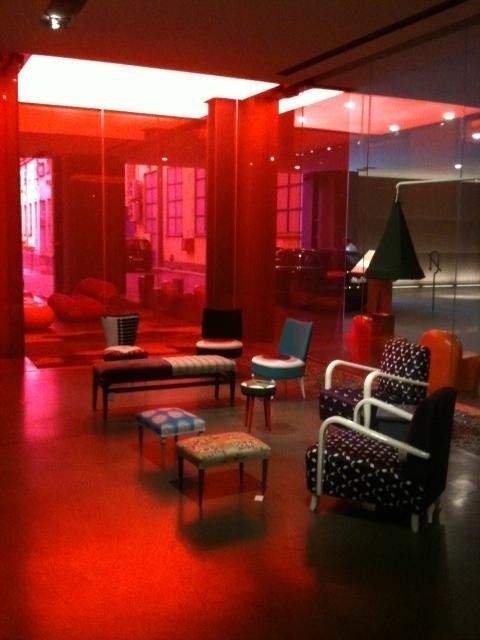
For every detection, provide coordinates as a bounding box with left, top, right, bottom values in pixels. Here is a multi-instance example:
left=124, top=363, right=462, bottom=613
left=316, top=335, right=432, bottom=430
left=49, top=275, right=118, bottom=322
left=24, top=289, right=56, bottom=325
left=418, top=328, right=480, bottom=396
left=304, top=385, right=460, bottom=534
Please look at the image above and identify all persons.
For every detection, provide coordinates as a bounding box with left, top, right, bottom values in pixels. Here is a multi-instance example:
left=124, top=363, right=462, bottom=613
left=341, top=236, right=358, bottom=252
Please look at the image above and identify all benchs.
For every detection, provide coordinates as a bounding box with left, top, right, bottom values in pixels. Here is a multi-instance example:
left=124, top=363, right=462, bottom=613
left=93, top=355, right=237, bottom=421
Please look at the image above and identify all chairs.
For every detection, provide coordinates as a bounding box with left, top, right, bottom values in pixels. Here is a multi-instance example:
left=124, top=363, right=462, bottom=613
left=101, top=312, right=149, bottom=360
left=251, top=316, right=315, bottom=400
left=196, top=307, right=244, bottom=358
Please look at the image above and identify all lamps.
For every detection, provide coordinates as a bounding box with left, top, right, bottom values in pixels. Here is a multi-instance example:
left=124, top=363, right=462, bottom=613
left=41, top=5, right=74, bottom=33
left=360, top=176, right=480, bottom=282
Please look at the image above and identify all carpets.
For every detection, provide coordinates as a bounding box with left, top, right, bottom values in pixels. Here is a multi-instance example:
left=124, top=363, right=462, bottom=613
left=21, top=288, right=205, bottom=369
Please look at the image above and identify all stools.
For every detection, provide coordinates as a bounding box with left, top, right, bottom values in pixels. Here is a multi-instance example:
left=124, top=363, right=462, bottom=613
left=136, top=407, right=206, bottom=468
left=241, top=380, right=277, bottom=434
left=175, top=431, right=273, bottom=505
